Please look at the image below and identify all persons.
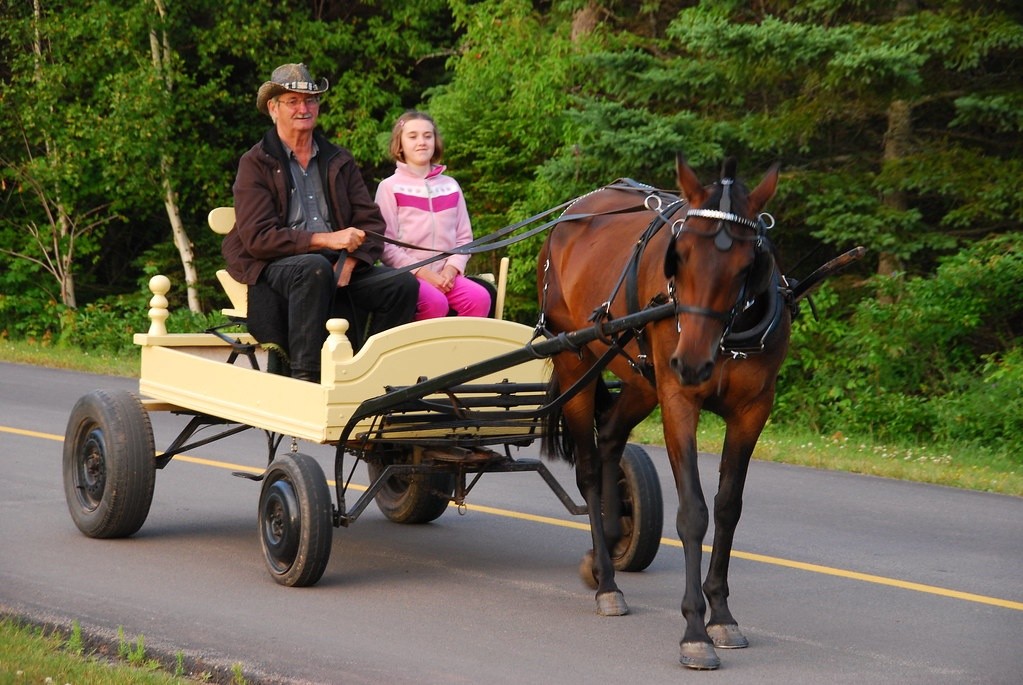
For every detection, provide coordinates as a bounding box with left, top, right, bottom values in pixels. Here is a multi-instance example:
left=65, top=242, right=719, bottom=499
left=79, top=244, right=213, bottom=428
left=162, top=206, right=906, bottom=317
left=221, top=63, right=420, bottom=383
left=374, top=112, right=491, bottom=321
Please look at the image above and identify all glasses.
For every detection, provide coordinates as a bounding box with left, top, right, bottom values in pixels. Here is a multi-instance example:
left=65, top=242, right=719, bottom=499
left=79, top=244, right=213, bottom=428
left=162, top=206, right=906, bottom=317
left=277, top=96, right=320, bottom=109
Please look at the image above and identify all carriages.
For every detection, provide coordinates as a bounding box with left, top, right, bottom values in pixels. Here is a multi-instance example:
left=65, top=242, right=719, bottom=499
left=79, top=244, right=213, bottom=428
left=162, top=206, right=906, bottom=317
left=62, top=157, right=866, bottom=668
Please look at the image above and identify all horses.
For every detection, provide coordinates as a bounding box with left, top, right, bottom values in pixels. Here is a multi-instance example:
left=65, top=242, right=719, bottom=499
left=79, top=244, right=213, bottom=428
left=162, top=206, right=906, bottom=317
left=536, top=149, right=793, bottom=671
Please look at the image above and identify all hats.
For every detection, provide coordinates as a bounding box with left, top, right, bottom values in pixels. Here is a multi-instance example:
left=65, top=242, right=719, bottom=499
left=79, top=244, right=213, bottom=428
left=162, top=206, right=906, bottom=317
left=257, top=62, right=329, bottom=116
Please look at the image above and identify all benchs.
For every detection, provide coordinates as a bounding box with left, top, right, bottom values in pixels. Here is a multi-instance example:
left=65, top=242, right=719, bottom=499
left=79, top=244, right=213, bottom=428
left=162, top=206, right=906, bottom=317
left=207, top=206, right=259, bottom=371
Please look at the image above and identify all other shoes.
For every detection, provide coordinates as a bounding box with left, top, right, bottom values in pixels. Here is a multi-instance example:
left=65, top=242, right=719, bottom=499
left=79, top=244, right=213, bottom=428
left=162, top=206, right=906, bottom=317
left=291, top=369, right=316, bottom=380
